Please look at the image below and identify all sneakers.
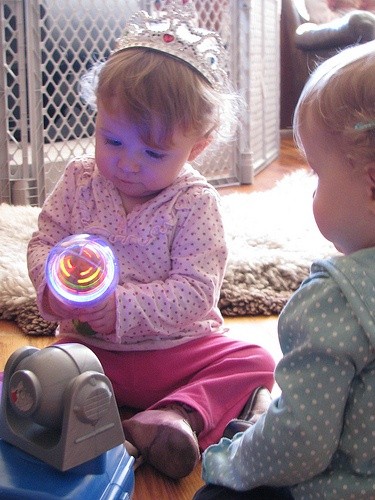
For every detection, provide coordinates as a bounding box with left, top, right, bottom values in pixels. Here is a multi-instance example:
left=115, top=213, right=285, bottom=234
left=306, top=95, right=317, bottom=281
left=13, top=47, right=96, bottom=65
left=238, top=384, right=274, bottom=424
left=222, top=418, right=253, bottom=439
left=122, top=409, right=200, bottom=480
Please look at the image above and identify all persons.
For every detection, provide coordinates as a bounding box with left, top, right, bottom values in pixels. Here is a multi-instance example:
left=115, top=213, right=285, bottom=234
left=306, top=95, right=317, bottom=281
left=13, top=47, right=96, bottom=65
left=28, top=40, right=275, bottom=480
left=192, top=41, right=375, bottom=500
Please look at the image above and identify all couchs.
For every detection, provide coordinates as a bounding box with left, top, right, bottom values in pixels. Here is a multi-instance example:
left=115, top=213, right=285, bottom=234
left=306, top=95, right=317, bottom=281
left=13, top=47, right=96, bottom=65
left=295, top=9, right=375, bottom=76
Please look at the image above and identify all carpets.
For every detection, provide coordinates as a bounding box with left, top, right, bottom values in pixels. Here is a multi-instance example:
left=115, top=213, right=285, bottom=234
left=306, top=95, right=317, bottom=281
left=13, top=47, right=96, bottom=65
left=0, top=167, right=327, bottom=338
